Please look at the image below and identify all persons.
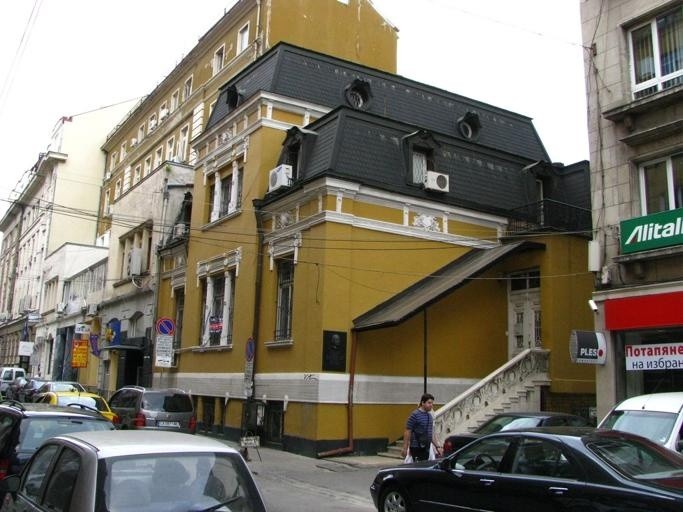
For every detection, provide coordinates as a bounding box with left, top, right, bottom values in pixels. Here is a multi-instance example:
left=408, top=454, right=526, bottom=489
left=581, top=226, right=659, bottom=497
left=400, top=393, right=443, bottom=464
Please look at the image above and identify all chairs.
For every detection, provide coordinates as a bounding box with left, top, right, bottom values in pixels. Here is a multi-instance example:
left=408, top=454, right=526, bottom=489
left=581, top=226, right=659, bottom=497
left=112, top=461, right=224, bottom=506
left=522, top=444, right=546, bottom=475
left=24, top=425, right=37, bottom=439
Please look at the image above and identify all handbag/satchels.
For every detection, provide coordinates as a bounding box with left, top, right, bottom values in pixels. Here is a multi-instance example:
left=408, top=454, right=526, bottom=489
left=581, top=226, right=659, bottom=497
left=415, top=430, right=428, bottom=449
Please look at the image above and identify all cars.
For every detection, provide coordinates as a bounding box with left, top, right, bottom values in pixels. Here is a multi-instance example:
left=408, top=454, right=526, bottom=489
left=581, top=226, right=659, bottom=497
left=596, top=392, right=683, bottom=480
left=0, top=366, right=268, bottom=511
left=370, top=425, right=683, bottom=512
left=441, top=412, right=595, bottom=459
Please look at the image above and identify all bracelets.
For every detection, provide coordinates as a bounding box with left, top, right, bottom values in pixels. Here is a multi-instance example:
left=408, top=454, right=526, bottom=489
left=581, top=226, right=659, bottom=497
left=435, top=445, right=442, bottom=450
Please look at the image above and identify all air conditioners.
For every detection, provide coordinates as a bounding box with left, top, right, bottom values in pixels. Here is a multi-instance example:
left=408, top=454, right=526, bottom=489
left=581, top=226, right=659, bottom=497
left=268, top=165, right=292, bottom=190
left=423, top=172, right=450, bottom=193
left=85, top=302, right=97, bottom=316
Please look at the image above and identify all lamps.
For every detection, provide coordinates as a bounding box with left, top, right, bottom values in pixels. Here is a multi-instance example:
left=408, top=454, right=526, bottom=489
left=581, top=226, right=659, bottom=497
left=587, top=240, right=602, bottom=273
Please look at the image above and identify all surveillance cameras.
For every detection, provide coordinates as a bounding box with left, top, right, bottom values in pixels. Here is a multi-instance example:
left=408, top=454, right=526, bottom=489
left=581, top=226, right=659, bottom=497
left=588, top=300, right=598, bottom=312
